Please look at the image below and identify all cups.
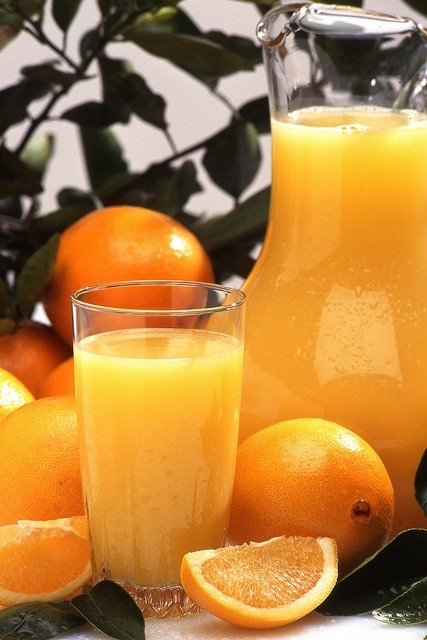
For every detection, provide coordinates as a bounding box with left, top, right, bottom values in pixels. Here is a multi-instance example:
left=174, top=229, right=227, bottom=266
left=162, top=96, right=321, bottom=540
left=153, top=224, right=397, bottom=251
left=203, top=0, right=427, bottom=557
left=69, top=280, right=249, bottom=621
